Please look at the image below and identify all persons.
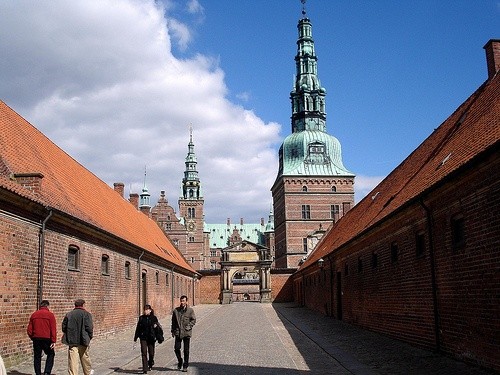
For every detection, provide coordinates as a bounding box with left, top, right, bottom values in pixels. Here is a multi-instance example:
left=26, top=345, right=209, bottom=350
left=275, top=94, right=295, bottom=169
left=171, top=295, right=196, bottom=372
left=134, top=304, right=164, bottom=375
left=26, top=300, right=57, bottom=375
left=61, top=299, right=95, bottom=375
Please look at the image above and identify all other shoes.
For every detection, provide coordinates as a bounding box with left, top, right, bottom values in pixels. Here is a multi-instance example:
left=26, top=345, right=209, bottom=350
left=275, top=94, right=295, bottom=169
left=183, top=368, right=187, bottom=371
left=178, top=358, right=183, bottom=369
left=143, top=371, right=147, bottom=375
left=90, top=369, right=94, bottom=375
left=149, top=366, right=152, bottom=370
